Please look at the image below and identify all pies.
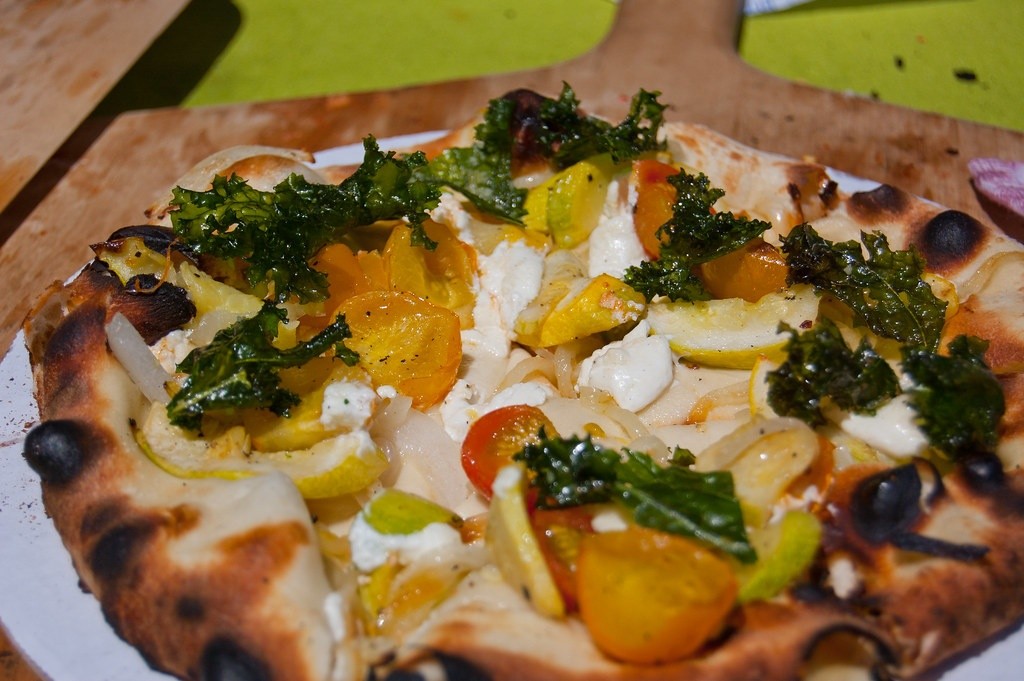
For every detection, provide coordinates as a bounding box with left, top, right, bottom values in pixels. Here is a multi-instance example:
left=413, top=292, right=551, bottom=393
left=27, top=88, right=1024, bottom=681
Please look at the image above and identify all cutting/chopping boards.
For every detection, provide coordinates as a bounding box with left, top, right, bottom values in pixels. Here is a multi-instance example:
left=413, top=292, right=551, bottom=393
left=0, top=0, right=1024, bottom=681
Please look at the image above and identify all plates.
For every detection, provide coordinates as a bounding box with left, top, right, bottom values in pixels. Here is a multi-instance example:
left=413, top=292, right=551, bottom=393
left=0, top=127, right=1024, bottom=681
